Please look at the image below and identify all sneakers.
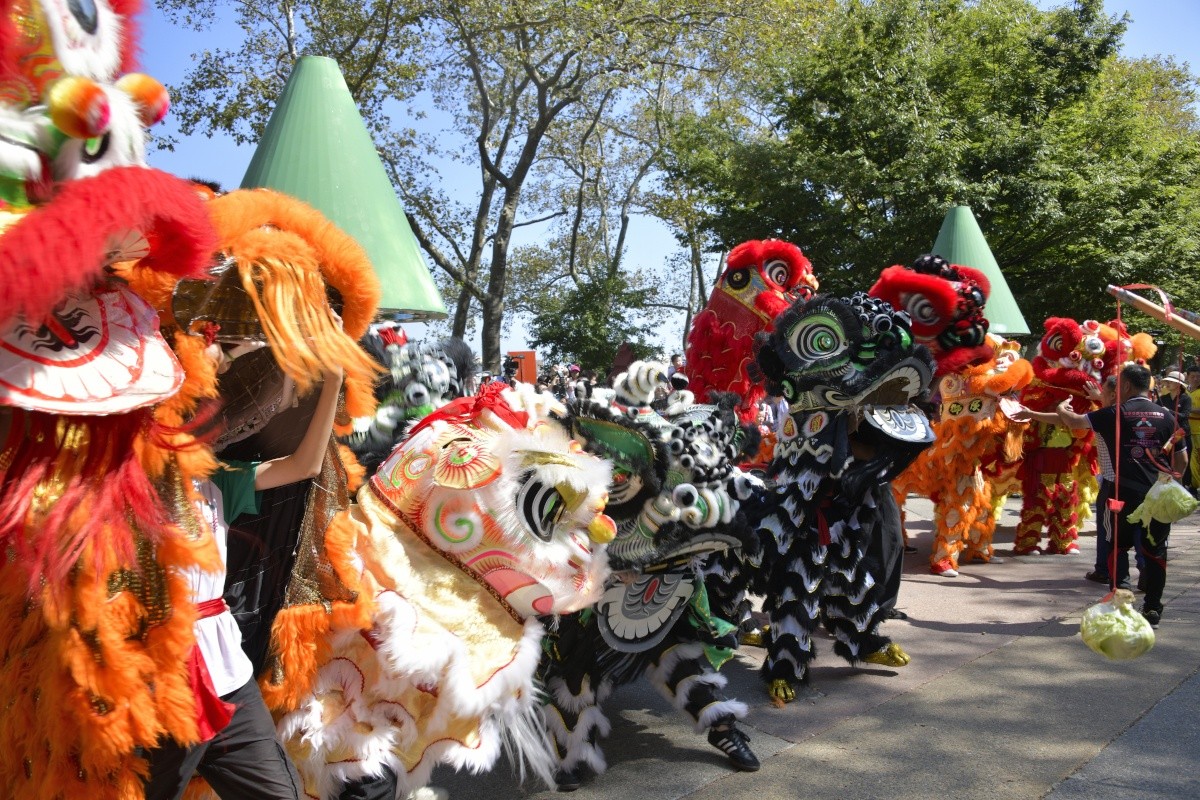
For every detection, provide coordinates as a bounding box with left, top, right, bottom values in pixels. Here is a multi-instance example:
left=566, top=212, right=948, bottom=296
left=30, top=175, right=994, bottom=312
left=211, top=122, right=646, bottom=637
left=935, top=563, right=959, bottom=576
left=973, top=555, right=1003, bottom=563
left=707, top=728, right=761, bottom=771
left=903, top=546, right=918, bottom=553
left=1142, top=604, right=1161, bottom=628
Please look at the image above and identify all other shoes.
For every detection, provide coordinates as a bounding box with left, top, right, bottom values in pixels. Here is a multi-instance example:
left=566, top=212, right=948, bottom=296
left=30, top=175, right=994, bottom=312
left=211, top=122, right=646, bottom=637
left=1110, top=584, right=1131, bottom=593
left=1087, top=571, right=1110, bottom=585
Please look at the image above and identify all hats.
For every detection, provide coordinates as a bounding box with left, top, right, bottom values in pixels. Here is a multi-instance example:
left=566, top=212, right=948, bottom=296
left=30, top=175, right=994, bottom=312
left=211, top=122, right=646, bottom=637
left=1162, top=371, right=1188, bottom=388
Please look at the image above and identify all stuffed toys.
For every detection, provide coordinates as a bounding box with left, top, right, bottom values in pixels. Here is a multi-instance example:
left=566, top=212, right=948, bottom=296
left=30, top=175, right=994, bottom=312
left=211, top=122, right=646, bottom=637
left=0, top=0, right=376, bottom=799
left=338, top=327, right=475, bottom=496
left=573, top=240, right=1156, bottom=800
left=257, top=379, right=616, bottom=800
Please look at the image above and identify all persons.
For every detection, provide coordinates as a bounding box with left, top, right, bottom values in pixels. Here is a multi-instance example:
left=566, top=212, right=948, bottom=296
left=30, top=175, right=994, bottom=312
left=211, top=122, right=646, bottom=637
left=138, top=311, right=342, bottom=800
left=1152, top=364, right=1200, bottom=500
left=1010, top=377, right=1145, bottom=591
left=477, top=359, right=598, bottom=406
left=1056, top=364, right=1188, bottom=629
left=666, top=354, right=686, bottom=377
